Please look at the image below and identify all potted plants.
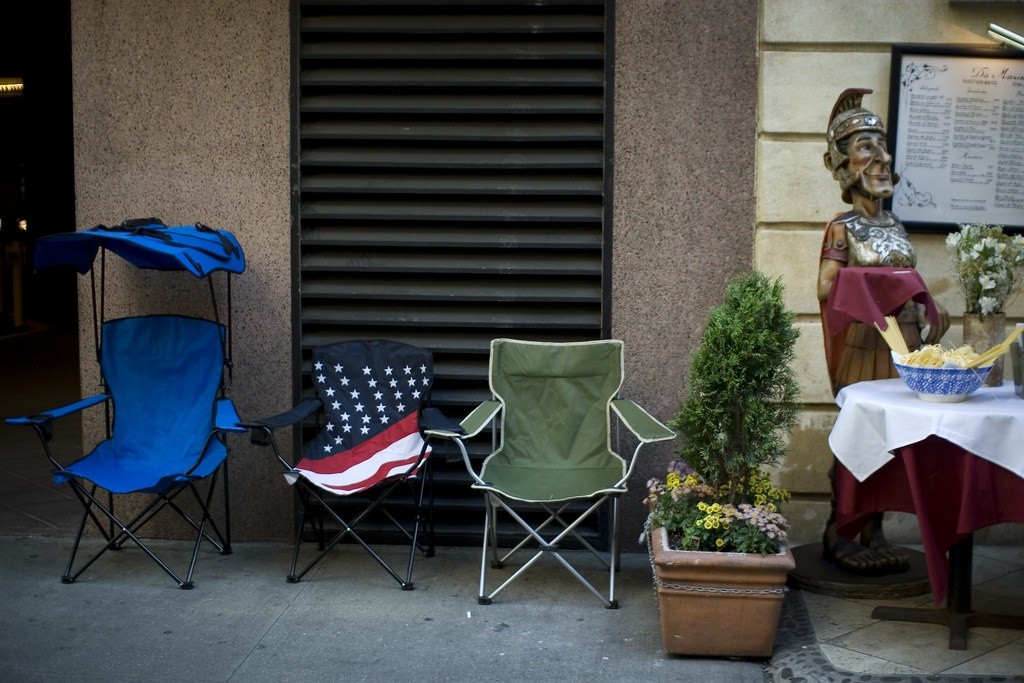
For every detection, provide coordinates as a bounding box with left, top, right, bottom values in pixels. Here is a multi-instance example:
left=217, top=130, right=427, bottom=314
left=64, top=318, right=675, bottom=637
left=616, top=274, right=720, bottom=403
left=648, top=270, right=802, bottom=658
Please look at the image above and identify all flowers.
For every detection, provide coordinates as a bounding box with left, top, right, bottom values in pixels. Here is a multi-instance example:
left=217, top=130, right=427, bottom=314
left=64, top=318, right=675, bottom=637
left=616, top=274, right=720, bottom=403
left=944, top=222, right=1024, bottom=317
left=637, top=468, right=791, bottom=556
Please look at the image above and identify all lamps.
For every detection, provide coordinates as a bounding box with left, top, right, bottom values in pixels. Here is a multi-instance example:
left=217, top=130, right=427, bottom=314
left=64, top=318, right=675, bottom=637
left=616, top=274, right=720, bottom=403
left=987, top=22, right=1024, bottom=51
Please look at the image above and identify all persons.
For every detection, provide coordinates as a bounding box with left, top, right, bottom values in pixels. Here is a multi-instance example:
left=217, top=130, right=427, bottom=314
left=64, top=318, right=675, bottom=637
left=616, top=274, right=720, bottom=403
left=818, top=89, right=950, bottom=575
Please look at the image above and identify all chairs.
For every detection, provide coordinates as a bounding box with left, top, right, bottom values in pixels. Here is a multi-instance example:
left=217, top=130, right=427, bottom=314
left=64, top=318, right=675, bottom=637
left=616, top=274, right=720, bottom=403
left=423, top=337, right=677, bottom=609
left=4, top=217, right=246, bottom=588
left=236, top=339, right=466, bottom=591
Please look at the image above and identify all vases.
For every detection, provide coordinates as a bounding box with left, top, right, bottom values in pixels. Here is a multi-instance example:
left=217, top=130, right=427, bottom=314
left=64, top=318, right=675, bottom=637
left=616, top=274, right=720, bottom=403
left=963, top=312, right=1004, bottom=388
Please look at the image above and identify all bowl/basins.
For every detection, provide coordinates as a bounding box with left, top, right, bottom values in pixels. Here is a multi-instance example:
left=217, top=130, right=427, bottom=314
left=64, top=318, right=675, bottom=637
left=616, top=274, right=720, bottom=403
left=892, top=361, right=995, bottom=403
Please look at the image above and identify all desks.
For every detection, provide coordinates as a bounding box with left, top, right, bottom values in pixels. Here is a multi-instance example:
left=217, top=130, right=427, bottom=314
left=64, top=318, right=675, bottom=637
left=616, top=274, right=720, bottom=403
left=828, top=378, right=1024, bottom=651
